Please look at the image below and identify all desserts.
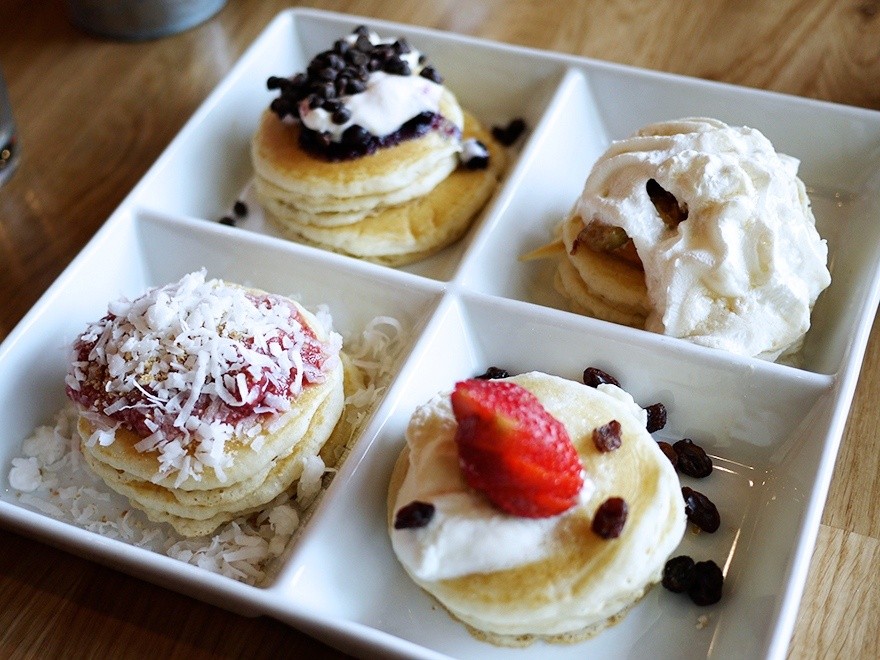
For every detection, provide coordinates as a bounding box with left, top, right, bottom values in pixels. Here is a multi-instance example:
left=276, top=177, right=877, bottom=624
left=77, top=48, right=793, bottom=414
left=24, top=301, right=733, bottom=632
left=519, top=117, right=833, bottom=357
left=383, top=371, right=690, bottom=647
left=247, top=24, right=507, bottom=264
left=61, top=265, right=348, bottom=538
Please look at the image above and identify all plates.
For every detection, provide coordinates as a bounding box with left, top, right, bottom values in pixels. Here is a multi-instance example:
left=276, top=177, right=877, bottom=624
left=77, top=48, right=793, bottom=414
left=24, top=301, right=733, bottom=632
left=0, top=5, right=880, bottom=660
